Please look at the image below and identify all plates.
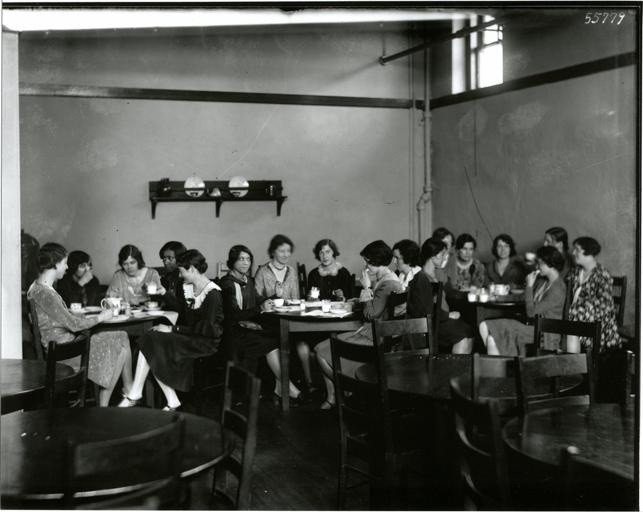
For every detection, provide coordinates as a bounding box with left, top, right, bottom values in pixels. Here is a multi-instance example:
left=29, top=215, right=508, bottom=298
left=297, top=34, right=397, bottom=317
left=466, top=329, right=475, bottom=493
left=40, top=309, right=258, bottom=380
left=184, top=175, right=206, bottom=197
left=228, top=176, right=250, bottom=198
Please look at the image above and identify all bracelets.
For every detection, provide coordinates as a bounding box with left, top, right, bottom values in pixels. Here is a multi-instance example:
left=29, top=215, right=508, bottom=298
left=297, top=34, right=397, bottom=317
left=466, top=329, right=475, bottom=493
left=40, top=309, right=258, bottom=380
left=171, top=325, right=177, bottom=334
left=364, top=286, right=372, bottom=290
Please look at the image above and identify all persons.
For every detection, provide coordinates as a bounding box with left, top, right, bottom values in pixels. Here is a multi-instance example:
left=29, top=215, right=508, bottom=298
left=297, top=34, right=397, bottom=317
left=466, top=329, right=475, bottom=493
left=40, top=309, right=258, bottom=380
left=142, top=241, right=187, bottom=325
left=104, top=244, right=160, bottom=307
left=312, top=239, right=403, bottom=416
left=304, top=239, right=354, bottom=356
left=55, top=250, right=99, bottom=307
left=115, top=248, right=224, bottom=412
left=26, top=242, right=135, bottom=408
left=253, top=234, right=320, bottom=398
left=219, top=244, right=312, bottom=407
left=563, top=237, right=624, bottom=354
left=392, top=227, right=572, bottom=357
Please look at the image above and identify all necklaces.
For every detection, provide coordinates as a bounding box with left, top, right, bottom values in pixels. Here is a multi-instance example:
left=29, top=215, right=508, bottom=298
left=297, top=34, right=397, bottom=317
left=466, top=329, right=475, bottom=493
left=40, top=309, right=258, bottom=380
left=193, top=278, right=210, bottom=296
left=271, top=261, right=285, bottom=271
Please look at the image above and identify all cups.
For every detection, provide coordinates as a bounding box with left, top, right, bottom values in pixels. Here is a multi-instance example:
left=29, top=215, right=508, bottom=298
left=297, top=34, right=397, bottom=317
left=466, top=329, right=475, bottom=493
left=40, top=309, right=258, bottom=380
left=70, top=284, right=158, bottom=325
left=270, top=287, right=330, bottom=318
left=465, top=282, right=512, bottom=302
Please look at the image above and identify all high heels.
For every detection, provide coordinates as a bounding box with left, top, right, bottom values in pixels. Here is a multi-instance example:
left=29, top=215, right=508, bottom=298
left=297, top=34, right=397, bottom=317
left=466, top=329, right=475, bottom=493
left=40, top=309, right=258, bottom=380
left=275, top=380, right=354, bottom=416
left=116, top=393, right=145, bottom=407
left=158, top=401, right=183, bottom=412
